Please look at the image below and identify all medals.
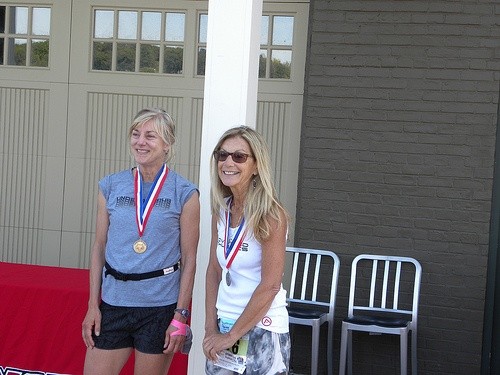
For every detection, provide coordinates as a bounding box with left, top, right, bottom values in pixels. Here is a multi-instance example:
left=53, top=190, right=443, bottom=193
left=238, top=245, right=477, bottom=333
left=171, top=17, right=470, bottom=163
left=133, top=238, right=147, bottom=253
left=226, top=272, right=231, bottom=286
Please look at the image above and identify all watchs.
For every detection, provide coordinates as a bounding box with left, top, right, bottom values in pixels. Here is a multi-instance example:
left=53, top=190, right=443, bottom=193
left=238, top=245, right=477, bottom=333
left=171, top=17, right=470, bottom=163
left=174, top=308, right=189, bottom=319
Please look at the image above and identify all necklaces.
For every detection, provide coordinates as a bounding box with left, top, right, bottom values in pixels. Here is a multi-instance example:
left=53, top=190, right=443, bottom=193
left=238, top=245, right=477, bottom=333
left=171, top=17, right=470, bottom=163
left=227, top=205, right=242, bottom=229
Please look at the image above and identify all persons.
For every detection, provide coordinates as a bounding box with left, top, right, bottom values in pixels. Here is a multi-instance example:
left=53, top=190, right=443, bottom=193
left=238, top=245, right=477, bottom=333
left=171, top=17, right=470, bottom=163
left=202, top=126, right=290, bottom=375
left=81, top=108, right=199, bottom=375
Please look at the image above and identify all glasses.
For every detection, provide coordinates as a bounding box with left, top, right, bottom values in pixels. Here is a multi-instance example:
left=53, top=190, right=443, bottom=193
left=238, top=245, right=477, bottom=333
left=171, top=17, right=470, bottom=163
left=213, top=151, right=255, bottom=163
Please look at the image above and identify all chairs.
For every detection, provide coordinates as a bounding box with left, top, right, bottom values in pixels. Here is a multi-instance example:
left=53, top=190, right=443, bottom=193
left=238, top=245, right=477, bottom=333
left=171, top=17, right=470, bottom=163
left=285, top=247, right=340, bottom=375
left=339, top=254, right=422, bottom=375
